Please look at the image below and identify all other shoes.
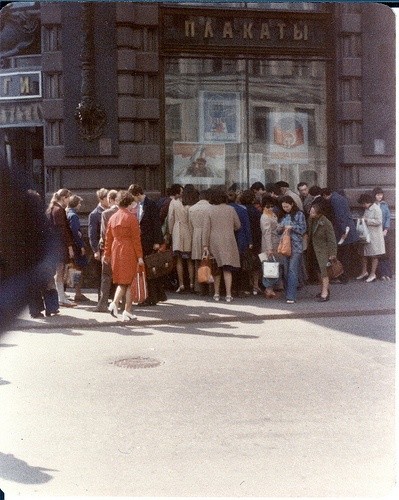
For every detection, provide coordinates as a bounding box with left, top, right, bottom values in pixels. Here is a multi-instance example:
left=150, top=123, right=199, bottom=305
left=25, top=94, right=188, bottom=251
left=31, top=312, right=44, bottom=318
left=176, top=284, right=185, bottom=293
left=365, top=274, right=377, bottom=283
left=46, top=309, right=59, bottom=314
left=265, top=290, right=280, bottom=299
left=212, top=294, right=220, bottom=301
left=356, top=272, right=369, bottom=279
left=59, top=301, right=77, bottom=307
left=332, top=280, right=347, bottom=285
left=75, top=294, right=89, bottom=302
left=238, top=290, right=250, bottom=298
left=287, top=299, right=295, bottom=304
left=253, top=287, right=261, bottom=296
left=316, top=288, right=330, bottom=302
left=380, top=276, right=390, bottom=280
left=225, top=296, right=233, bottom=303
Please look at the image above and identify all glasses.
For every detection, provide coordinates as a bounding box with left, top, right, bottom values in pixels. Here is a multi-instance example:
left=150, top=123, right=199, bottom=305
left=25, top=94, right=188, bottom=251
left=264, top=206, right=275, bottom=209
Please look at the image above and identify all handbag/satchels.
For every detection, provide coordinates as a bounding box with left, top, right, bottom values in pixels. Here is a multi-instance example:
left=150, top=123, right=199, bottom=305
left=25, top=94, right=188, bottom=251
left=198, top=249, right=212, bottom=269
left=130, top=264, right=148, bottom=302
left=262, top=253, right=279, bottom=278
left=64, top=257, right=82, bottom=287
left=354, top=218, right=370, bottom=245
left=277, top=230, right=291, bottom=257
left=144, top=249, right=174, bottom=279
left=327, top=257, right=344, bottom=278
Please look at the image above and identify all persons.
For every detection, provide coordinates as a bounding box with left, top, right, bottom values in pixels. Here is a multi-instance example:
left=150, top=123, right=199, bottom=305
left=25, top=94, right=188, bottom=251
left=27, top=188, right=60, bottom=318
left=46, top=181, right=391, bottom=321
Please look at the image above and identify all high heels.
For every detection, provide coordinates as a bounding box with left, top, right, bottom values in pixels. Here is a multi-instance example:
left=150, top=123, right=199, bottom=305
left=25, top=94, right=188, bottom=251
left=108, top=302, right=118, bottom=318
left=122, top=310, right=137, bottom=321
